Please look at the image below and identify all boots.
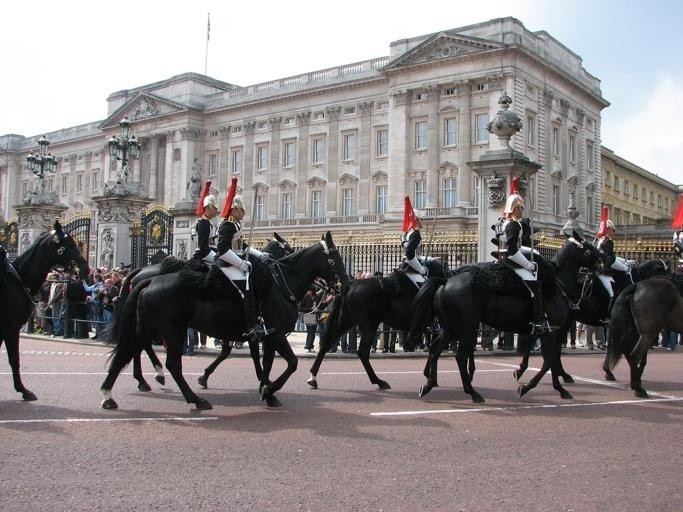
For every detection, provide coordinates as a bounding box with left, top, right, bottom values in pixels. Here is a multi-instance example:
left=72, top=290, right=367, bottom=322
left=608, top=297, right=616, bottom=318
left=529, top=296, right=561, bottom=335
left=243, top=290, right=276, bottom=337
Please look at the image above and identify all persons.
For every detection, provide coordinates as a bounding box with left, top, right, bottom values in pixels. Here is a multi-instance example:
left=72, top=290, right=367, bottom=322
left=498, top=193, right=560, bottom=337
left=214, top=196, right=276, bottom=341
left=186, top=195, right=218, bottom=265
left=26, top=261, right=251, bottom=352
left=671, top=226, right=683, bottom=273
left=400, top=207, right=443, bottom=338
left=592, top=218, right=636, bottom=315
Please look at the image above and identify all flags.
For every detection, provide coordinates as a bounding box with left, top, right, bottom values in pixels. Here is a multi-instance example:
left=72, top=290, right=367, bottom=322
left=208, top=15, right=210, bottom=40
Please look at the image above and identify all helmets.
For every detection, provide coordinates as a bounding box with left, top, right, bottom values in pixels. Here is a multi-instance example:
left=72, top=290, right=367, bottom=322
left=413, top=208, right=423, bottom=219
left=203, top=194, right=218, bottom=209
left=600, top=219, right=615, bottom=231
left=231, top=195, right=246, bottom=210
left=504, top=194, right=525, bottom=214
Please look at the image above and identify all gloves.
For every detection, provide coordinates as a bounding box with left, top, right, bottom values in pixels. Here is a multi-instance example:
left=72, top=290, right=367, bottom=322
left=405, top=256, right=427, bottom=275
left=518, top=246, right=541, bottom=256
left=202, top=250, right=217, bottom=264
left=421, top=256, right=442, bottom=265
left=244, top=247, right=268, bottom=264
left=507, top=250, right=536, bottom=272
left=219, top=250, right=250, bottom=272
left=611, top=256, right=635, bottom=272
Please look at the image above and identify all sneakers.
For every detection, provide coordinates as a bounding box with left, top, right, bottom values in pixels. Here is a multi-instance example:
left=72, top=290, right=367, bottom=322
left=390, top=347, right=395, bottom=353
left=371, top=348, right=376, bottom=353
left=570, top=344, right=576, bottom=349
left=73, top=334, right=97, bottom=340
left=404, top=347, right=414, bottom=352
left=382, top=348, right=388, bottom=353
left=193, top=345, right=198, bottom=349
left=329, top=349, right=357, bottom=354
left=200, top=344, right=206, bottom=349
left=562, top=344, right=566, bottom=348
left=580, top=342, right=606, bottom=350
left=20, top=326, right=51, bottom=336
left=216, top=343, right=243, bottom=349
left=650, top=344, right=670, bottom=353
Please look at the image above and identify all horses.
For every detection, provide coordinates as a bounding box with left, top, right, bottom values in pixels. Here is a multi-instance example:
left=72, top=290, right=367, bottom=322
left=306, top=233, right=612, bottom=404
left=0, top=219, right=91, bottom=401
left=557, top=252, right=681, bottom=398
left=93, top=231, right=348, bottom=409
